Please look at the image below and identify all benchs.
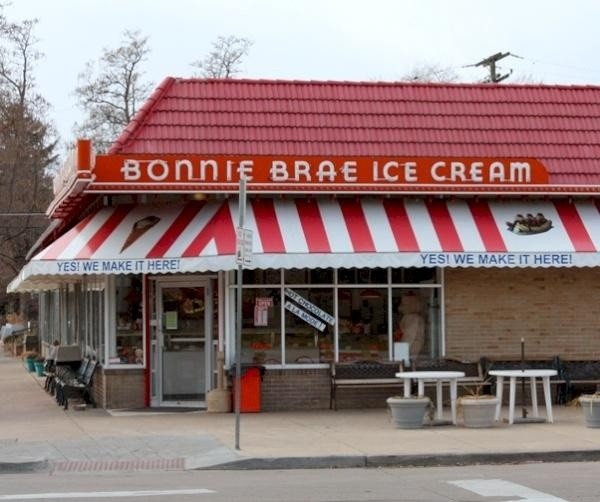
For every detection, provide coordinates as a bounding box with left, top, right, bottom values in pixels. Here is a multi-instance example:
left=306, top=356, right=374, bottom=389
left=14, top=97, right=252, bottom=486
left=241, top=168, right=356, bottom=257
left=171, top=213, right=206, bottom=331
left=329, top=358, right=600, bottom=412
left=43, top=355, right=99, bottom=411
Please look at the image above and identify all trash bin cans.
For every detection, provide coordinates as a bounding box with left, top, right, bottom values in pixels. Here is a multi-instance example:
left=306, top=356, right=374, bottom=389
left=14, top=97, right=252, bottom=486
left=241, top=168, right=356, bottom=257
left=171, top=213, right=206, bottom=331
left=23, top=335, right=39, bottom=352
left=225, top=363, right=267, bottom=413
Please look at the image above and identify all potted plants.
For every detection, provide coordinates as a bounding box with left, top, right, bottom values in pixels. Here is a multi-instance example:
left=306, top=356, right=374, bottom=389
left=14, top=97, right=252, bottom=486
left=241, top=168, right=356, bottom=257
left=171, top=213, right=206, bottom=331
left=34, top=356, right=46, bottom=376
left=26, top=352, right=35, bottom=371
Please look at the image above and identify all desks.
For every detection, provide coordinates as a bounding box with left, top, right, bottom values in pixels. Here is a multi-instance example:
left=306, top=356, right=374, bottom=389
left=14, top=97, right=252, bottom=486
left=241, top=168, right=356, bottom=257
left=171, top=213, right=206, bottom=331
left=488, top=369, right=558, bottom=423
left=395, top=371, right=465, bottom=425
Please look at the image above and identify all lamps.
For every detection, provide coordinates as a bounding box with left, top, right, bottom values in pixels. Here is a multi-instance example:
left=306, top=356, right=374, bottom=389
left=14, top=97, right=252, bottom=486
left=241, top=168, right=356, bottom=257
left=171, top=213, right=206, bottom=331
left=359, top=269, right=381, bottom=297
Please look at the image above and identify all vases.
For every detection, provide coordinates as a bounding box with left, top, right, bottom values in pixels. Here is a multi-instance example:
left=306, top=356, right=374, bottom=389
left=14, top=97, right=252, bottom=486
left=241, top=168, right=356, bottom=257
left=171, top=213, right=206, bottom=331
left=460, top=398, right=499, bottom=428
left=386, top=396, right=429, bottom=428
left=578, top=395, right=600, bottom=428
